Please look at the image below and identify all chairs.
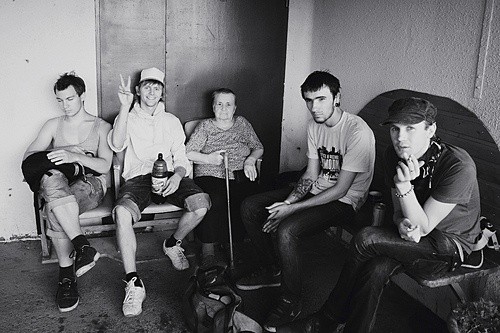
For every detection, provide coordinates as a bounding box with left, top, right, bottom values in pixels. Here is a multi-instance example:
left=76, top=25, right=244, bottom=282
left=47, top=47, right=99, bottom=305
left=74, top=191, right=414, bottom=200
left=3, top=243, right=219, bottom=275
left=37, top=119, right=263, bottom=259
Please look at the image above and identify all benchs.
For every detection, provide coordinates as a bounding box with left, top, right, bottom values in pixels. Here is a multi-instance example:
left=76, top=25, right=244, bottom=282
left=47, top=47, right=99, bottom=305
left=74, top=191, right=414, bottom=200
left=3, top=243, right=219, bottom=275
left=282, top=90, right=500, bottom=304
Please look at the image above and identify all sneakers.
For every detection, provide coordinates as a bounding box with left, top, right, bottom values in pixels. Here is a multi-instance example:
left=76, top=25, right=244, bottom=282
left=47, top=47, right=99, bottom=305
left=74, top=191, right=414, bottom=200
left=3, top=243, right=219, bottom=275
left=56, top=272, right=79, bottom=312
left=236, top=269, right=282, bottom=290
left=121, top=277, right=147, bottom=317
left=69, top=245, right=101, bottom=277
left=163, top=239, right=189, bottom=270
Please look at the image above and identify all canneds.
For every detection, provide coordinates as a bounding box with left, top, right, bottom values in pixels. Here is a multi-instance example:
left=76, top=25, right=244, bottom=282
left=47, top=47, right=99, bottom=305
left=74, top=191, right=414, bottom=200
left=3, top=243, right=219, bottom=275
left=371, top=202, right=386, bottom=227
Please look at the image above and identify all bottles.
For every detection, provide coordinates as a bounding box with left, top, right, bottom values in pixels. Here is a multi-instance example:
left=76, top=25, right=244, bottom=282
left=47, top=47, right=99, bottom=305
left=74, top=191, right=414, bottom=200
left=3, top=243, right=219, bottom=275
left=151, top=153, right=169, bottom=204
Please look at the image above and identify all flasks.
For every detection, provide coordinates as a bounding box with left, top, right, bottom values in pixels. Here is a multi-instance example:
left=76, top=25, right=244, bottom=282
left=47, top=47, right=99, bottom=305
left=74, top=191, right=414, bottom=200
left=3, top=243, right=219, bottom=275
left=363, top=189, right=389, bottom=227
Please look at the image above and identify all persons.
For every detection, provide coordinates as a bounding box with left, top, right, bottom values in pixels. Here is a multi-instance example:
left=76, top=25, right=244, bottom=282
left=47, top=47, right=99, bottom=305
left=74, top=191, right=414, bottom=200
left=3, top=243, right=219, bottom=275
left=235, top=70, right=377, bottom=332
left=23, top=73, right=113, bottom=313
left=277, top=98, right=482, bottom=333
left=186, top=88, right=264, bottom=271
left=107, top=67, right=212, bottom=317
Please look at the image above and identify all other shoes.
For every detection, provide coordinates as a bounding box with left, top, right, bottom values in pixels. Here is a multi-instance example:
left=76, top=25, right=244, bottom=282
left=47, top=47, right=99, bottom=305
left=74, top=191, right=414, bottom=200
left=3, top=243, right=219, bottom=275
left=201, top=255, right=227, bottom=269
left=263, top=297, right=301, bottom=332
left=303, top=317, right=320, bottom=333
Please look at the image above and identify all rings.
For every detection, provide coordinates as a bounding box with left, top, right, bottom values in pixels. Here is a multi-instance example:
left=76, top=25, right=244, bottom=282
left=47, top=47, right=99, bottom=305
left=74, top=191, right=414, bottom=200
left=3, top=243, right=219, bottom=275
left=410, top=171, right=413, bottom=174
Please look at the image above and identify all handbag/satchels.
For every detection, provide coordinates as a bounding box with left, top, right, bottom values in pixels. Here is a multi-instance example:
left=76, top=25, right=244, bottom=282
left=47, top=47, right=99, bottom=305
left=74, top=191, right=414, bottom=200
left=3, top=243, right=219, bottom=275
left=183, top=255, right=262, bottom=333
left=21, top=151, right=102, bottom=212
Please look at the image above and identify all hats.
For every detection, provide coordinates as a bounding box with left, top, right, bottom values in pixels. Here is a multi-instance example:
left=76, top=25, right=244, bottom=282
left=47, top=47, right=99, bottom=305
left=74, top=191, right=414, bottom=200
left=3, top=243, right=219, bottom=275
left=139, top=67, right=165, bottom=85
left=382, top=97, right=438, bottom=126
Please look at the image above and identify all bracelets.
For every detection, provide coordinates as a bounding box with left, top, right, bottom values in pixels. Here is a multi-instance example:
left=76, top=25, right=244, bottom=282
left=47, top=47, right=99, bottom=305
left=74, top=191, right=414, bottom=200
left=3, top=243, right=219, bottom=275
left=394, top=185, right=415, bottom=198
left=284, top=198, right=292, bottom=205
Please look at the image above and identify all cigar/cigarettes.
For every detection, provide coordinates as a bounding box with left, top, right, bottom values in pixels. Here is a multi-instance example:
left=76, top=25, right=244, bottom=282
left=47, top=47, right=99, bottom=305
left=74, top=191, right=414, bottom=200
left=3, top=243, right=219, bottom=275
left=408, top=157, right=412, bottom=162
